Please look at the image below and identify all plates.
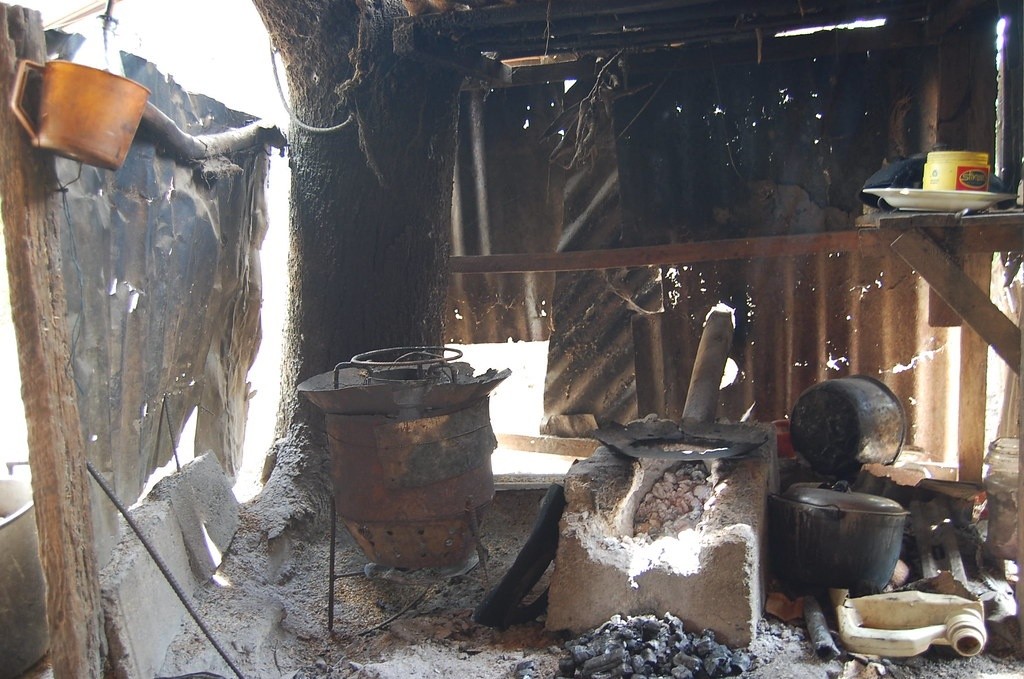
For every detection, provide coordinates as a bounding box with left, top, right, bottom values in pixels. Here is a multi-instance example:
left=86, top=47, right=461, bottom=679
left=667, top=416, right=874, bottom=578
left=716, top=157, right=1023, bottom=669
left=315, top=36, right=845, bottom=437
left=861, top=188, right=1015, bottom=212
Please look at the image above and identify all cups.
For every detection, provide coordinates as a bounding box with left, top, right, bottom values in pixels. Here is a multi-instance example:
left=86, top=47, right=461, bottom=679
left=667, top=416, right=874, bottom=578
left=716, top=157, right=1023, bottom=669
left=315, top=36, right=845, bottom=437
left=11, top=60, right=152, bottom=170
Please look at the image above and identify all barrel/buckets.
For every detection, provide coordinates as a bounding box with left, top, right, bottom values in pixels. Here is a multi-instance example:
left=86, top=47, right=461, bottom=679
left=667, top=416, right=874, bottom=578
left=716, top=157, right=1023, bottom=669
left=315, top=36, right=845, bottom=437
left=0, top=462, right=76, bottom=679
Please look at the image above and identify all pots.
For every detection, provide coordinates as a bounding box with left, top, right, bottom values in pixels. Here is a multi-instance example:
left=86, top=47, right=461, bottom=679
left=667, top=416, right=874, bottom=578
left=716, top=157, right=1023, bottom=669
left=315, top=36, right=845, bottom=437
left=856, top=157, right=1008, bottom=209
left=791, top=374, right=907, bottom=477
left=768, top=481, right=912, bottom=590
